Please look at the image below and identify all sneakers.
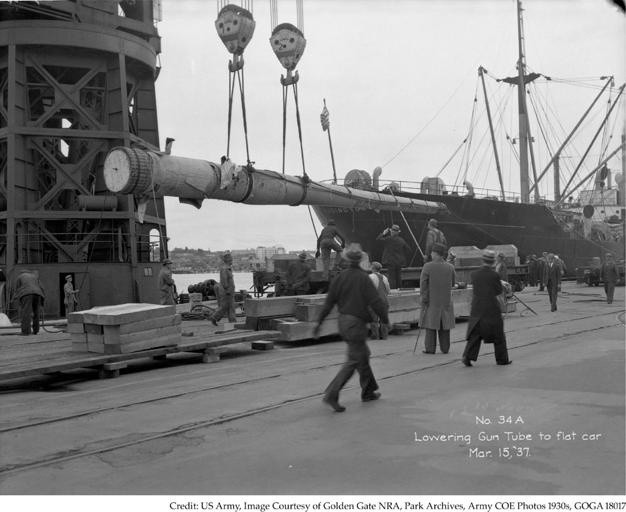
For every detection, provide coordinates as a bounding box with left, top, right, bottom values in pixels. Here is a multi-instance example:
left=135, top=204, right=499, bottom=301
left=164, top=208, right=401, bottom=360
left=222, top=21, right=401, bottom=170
left=607, top=298, right=612, bottom=304
left=496, top=360, right=513, bottom=366
left=229, top=318, right=238, bottom=323
left=210, top=316, right=219, bottom=327
left=423, top=349, right=435, bottom=355
left=554, top=304, right=558, bottom=311
left=441, top=347, right=449, bottom=354
left=32, top=330, right=39, bottom=335
left=462, top=358, right=473, bottom=367
left=20, top=332, right=31, bottom=336
left=323, top=394, right=346, bottom=413
left=361, top=390, right=381, bottom=402
left=551, top=308, right=554, bottom=312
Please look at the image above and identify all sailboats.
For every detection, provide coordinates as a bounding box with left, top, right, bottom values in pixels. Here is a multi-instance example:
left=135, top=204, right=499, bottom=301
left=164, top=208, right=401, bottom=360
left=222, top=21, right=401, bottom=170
left=104, top=0, right=626, bottom=292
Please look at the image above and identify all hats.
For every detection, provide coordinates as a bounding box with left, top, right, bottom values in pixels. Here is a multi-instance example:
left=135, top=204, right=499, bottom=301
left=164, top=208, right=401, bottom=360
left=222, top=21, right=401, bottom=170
left=65, top=275, right=72, bottom=279
left=427, top=218, right=440, bottom=227
left=480, top=250, right=499, bottom=261
left=339, top=242, right=368, bottom=262
left=163, top=259, right=171, bottom=263
left=298, top=253, right=305, bottom=258
left=327, top=219, right=336, bottom=225
left=427, top=243, right=451, bottom=253
left=390, top=224, right=402, bottom=233
left=371, top=262, right=383, bottom=270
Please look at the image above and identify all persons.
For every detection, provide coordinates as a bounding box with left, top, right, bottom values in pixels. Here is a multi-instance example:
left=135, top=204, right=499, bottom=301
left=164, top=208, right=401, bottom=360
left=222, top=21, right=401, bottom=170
left=312, top=250, right=393, bottom=412
left=314, top=218, right=342, bottom=280
left=496, top=248, right=618, bottom=313
left=211, top=252, right=235, bottom=327
left=419, top=241, right=456, bottom=353
left=62, top=274, right=78, bottom=316
left=157, top=260, right=176, bottom=304
left=375, top=225, right=412, bottom=289
left=13, top=270, right=44, bottom=336
left=285, top=252, right=311, bottom=295
left=460, top=250, right=512, bottom=368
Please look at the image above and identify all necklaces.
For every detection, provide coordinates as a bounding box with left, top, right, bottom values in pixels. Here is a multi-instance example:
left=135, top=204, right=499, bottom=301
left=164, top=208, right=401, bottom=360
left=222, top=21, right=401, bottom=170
left=425, top=218, right=446, bottom=258
